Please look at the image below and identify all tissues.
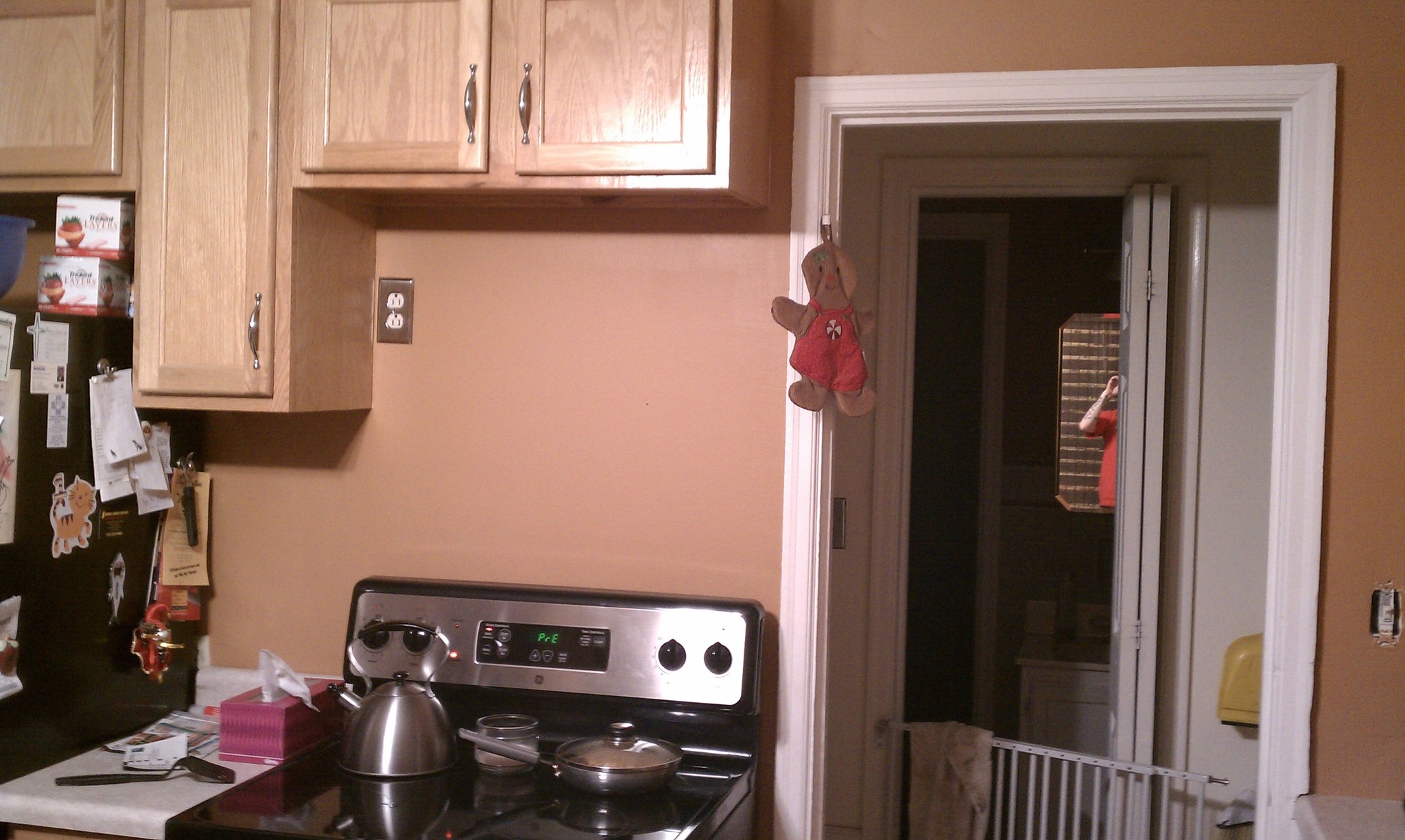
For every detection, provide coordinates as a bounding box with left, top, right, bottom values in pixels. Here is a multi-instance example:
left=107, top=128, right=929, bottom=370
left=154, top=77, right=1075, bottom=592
left=214, top=645, right=348, bottom=767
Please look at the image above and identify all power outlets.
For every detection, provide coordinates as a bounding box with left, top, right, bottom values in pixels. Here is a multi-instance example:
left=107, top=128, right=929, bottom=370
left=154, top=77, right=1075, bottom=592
left=376, top=277, right=414, bottom=345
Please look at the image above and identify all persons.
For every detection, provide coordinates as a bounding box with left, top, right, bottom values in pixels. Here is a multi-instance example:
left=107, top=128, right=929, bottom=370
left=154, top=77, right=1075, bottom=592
left=1078, top=375, right=1117, bottom=508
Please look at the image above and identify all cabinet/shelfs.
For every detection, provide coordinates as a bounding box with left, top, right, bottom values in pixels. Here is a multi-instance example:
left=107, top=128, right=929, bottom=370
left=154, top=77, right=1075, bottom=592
left=290, top=1, right=778, bottom=207
left=0, top=0, right=135, bottom=195
left=132, top=1, right=373, bottom=415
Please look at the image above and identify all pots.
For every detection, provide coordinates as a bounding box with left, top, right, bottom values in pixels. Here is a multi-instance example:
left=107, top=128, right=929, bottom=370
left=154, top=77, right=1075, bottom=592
left=458, top=723, right=684, bottom=792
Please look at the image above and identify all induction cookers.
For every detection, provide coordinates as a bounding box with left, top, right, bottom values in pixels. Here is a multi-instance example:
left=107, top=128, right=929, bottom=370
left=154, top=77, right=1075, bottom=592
left=165, top=574, right=767, bottom=839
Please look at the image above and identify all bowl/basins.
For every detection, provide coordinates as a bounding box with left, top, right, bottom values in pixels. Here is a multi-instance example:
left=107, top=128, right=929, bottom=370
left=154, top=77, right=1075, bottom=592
left=0, top=214, right=36, bottom=298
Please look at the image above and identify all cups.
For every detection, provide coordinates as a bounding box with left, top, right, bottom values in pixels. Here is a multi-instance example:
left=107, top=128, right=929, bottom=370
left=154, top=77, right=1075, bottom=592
left=475, top=714, right=538, bottom=775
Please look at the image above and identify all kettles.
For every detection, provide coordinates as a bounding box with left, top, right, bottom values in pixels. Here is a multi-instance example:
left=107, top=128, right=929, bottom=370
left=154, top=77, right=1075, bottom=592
left=327, top=620, right=457, bottom=776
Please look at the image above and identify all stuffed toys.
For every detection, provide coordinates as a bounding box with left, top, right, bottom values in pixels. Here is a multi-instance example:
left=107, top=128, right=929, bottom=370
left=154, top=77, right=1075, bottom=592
left=772, top=239, right=880, bottom=417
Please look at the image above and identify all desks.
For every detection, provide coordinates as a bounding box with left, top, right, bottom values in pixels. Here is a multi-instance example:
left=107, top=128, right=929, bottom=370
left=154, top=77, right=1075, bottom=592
left=0, top=661, right=345, bottom=837
